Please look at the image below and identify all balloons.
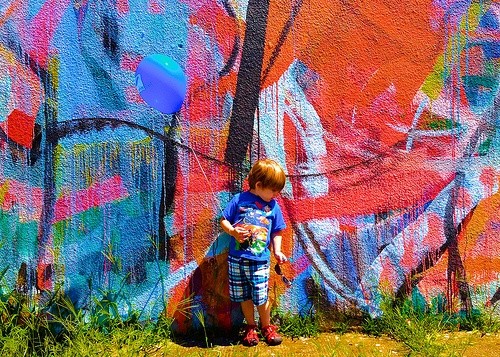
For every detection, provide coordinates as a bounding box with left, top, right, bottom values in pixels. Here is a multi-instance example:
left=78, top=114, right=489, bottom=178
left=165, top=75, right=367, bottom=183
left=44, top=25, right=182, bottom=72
left=134, top=54, right=187, bottom=115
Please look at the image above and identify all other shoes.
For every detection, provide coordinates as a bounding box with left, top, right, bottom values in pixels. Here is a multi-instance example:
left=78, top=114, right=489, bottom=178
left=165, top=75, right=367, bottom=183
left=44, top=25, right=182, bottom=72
left=262, top=325, right=283, bottom=345
left=243, top=324, right=259, bottom=346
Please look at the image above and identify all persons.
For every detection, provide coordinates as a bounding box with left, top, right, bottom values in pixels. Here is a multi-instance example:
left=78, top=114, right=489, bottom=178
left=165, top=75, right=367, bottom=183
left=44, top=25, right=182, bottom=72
left=219, top=159, right=285, bottom=344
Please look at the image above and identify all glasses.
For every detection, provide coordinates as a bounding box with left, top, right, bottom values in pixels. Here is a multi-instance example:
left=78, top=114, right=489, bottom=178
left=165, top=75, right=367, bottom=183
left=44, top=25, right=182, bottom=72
left=274, top=263, right=291, bottom=285
